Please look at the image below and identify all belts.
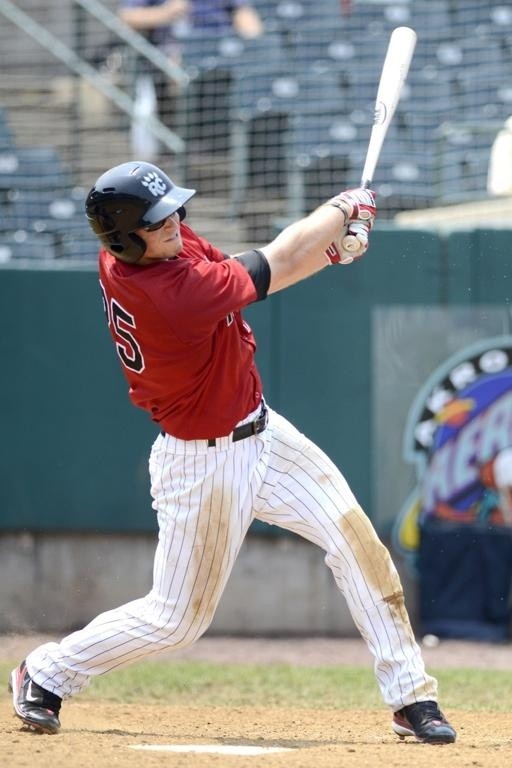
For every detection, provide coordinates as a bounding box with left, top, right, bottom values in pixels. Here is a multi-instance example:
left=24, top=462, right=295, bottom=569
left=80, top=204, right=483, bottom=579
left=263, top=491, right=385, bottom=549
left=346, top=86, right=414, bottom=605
left=208, top=400, right=273, bottom=447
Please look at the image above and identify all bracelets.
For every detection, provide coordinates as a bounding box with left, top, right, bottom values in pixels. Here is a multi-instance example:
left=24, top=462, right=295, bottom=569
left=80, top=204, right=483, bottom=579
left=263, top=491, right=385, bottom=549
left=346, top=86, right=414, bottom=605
left=328, top=202, right=348, bottom=226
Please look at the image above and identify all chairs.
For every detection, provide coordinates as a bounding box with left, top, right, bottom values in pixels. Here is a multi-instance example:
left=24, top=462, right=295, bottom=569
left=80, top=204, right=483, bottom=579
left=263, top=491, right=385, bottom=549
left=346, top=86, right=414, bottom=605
left=0, top=109, right=102, bottom=272
left=232, top=1, right=512, bottom=207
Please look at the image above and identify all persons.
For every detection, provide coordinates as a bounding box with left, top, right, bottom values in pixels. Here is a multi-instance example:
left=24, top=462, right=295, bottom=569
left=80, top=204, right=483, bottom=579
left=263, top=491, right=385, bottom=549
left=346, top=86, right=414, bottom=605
left=120, top=2, right=264, bottom=193
left=12, top=161, right=455, bottom=745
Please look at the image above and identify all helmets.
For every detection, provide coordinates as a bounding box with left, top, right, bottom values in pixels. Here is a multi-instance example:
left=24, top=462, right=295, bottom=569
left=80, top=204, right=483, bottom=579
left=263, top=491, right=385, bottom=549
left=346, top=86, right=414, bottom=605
left=85, top=161, right=200, bottom=263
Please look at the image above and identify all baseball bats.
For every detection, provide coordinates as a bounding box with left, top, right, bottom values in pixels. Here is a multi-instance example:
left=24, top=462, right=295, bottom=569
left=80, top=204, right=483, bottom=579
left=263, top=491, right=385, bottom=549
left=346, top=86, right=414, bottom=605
left=340, top=26, right=418, bottom=253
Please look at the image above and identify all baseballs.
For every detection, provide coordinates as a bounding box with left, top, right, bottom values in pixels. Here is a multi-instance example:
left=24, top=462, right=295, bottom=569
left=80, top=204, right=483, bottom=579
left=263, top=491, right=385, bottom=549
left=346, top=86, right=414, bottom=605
left=424, top=634, right=438, bottom=647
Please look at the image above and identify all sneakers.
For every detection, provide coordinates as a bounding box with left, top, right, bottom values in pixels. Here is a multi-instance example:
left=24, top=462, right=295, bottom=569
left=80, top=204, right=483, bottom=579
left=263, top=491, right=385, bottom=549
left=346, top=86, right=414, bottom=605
left=8, top=659, right=63, bottom=736
left=391, top=700, right=458, bottom=748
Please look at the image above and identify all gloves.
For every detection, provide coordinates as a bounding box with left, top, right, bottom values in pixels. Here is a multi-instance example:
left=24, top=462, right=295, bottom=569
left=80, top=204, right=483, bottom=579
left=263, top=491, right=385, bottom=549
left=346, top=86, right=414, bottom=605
left=326, top=186, right=382, bottom=225
left=324, top=225, right=369, bottom=268
